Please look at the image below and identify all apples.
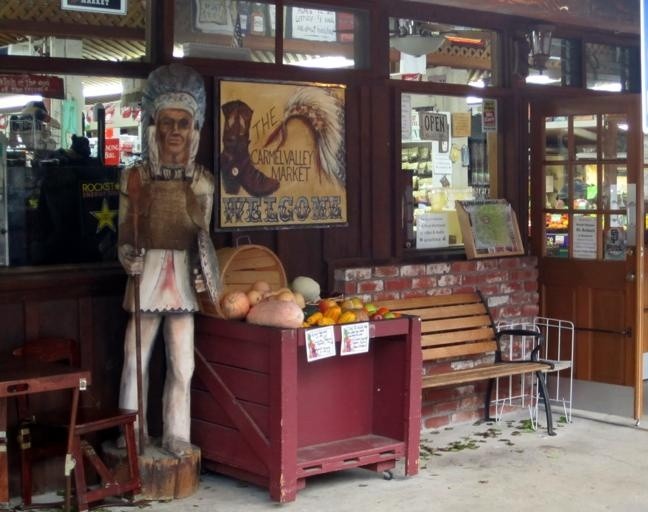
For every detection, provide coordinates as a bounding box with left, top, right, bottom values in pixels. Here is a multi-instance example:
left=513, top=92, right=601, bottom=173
left=365, top=303, right=402, bottom=321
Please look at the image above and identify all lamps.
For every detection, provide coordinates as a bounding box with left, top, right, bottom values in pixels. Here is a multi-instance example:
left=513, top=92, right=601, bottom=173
left=510, top=23, right=556, bottom=73
left=332, top=4, right=490, bottom=37
left=392, top=34, right=446, bottom=58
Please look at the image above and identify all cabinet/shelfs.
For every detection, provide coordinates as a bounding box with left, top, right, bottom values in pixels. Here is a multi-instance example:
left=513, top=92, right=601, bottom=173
left=492, top=314, right=574, bottom=424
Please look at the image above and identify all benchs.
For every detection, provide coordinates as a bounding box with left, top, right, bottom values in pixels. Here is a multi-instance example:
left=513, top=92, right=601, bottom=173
left=371, top=290, right=556, bottom=436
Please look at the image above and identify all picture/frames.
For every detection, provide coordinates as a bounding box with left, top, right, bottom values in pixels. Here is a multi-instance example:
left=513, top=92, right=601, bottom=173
left=214, top=73, right=350, bottom=233
left=60, top=0, right=129, bottom=16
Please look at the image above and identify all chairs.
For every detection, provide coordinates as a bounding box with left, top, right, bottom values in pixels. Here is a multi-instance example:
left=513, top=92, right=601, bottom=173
left=9, top=332, right=142, bottom=511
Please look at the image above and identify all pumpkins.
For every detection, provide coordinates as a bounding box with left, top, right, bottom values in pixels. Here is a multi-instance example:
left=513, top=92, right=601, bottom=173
left=221, top=276, right=369, bottom=329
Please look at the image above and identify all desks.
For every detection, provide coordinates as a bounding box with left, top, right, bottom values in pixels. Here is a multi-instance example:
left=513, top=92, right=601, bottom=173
left=190, top=312, right=423, bottom=504
left=1, top=350, right=93, bottom=511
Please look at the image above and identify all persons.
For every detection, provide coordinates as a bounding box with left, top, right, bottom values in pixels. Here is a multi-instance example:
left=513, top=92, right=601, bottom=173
left=52, top=132, right=92, bottom=161
left=112, top=63, right=231, bottom=466
